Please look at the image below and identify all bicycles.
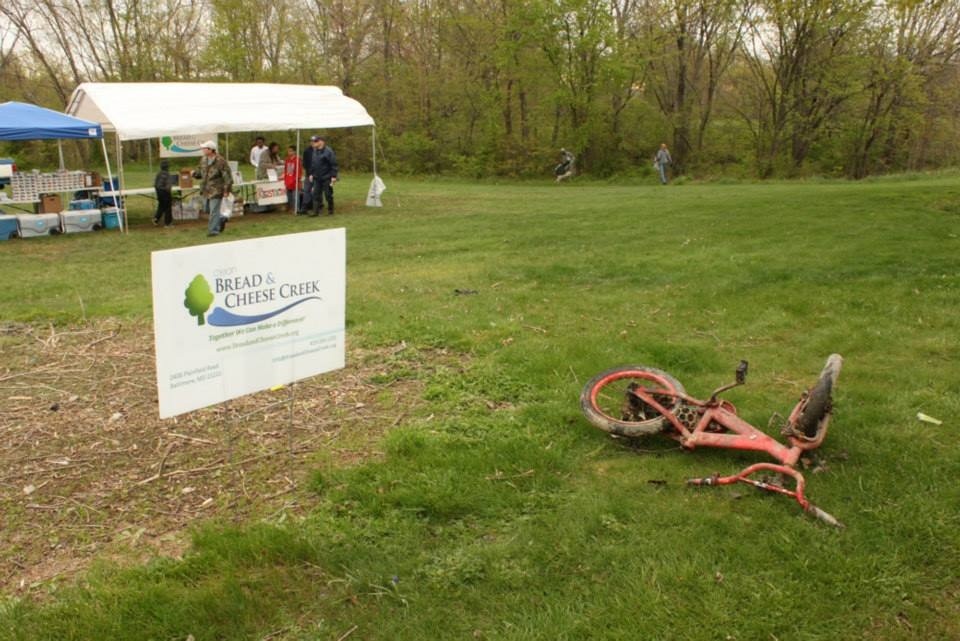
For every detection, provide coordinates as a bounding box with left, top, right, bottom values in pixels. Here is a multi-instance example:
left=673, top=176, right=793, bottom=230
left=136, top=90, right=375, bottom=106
left=579, top=352, right=846, bottom=531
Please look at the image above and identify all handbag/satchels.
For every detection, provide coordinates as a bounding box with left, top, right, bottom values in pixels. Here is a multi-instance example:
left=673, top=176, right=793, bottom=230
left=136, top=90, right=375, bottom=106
left=219, top=193, right=234, bottom=220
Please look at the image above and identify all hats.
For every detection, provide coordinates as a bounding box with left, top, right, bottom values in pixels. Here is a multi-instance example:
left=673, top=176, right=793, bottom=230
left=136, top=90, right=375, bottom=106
left=310, top=135, right=323, bottom=142
left=200, top=140, right=217, bottom=152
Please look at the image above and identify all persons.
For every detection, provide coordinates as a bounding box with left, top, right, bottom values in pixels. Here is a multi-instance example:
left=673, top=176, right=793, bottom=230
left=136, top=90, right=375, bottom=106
left=150, top=162, right=175, bottom=229
left=278, top=145, right=303, bottom=214
left=258, top=142, right=285, bottom=180
left=199, top=141, right=233, bottom=236
left=656, top=143, right=672, bottom=185
left=249, top=136, right=268, bottom=169
left=552, top=148, right=577, bottom=183
left=298, top=134, right=338, bottom=217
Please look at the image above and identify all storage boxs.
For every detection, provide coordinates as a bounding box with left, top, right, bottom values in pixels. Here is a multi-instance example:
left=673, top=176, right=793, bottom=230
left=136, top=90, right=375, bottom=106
left=172, top=206, right=199, bottom=220
left=0, top=176, right=123, bottom=241
left=179, top=166, right=193, bottom=187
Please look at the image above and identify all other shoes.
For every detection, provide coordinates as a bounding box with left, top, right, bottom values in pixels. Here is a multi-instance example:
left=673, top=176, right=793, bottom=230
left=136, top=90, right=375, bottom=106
left=208, top=232, right=219, bottom=237
left=152, top=218, right=159, bottom=227
left=310, top=212, right=319, bottom=217
left=164, top=225, right=176, bottom=229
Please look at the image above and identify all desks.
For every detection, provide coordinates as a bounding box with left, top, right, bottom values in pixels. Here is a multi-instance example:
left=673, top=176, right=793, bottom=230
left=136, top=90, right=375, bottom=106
left=96, top=179, right=283, bottom=221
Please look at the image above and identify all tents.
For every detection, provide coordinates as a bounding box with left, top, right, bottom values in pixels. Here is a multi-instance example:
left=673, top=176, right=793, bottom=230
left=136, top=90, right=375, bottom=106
left=0, top=101, right=123, bottom=233
left=55, top=82, right=375, bottom=232
